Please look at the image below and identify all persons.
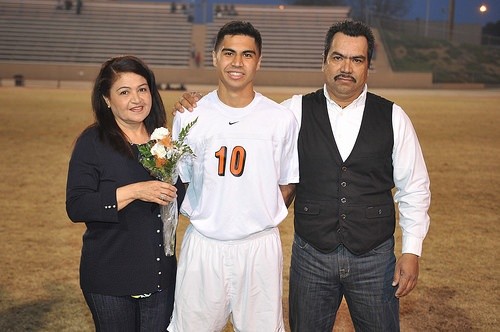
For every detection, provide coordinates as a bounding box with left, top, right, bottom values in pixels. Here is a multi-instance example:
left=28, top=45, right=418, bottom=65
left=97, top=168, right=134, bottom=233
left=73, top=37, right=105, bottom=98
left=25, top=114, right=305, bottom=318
left=55, top=0, right=83, bottom=15
left=66, top=55, right=186, bottom=332
left=167, top=21, right=299, bottom=332
left=171, top=18, right=431, bottom=332
left=169, top=1, right=237, bottom=16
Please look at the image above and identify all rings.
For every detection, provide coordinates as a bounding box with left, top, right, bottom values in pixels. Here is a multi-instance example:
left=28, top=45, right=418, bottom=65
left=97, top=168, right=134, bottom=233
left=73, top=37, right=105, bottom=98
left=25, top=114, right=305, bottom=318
left=160, top=193, right=164, bottom=200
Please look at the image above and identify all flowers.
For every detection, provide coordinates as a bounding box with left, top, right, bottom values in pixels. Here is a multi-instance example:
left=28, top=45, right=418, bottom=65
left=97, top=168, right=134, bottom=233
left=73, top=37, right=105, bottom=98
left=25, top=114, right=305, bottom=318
left=136, top=116, right=200, bottom=254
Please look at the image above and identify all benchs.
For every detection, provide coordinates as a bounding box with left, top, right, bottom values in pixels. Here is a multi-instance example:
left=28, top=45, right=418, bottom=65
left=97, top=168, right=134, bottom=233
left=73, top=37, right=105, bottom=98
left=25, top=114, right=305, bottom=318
left=0, top=0, right=377, bottom=73
